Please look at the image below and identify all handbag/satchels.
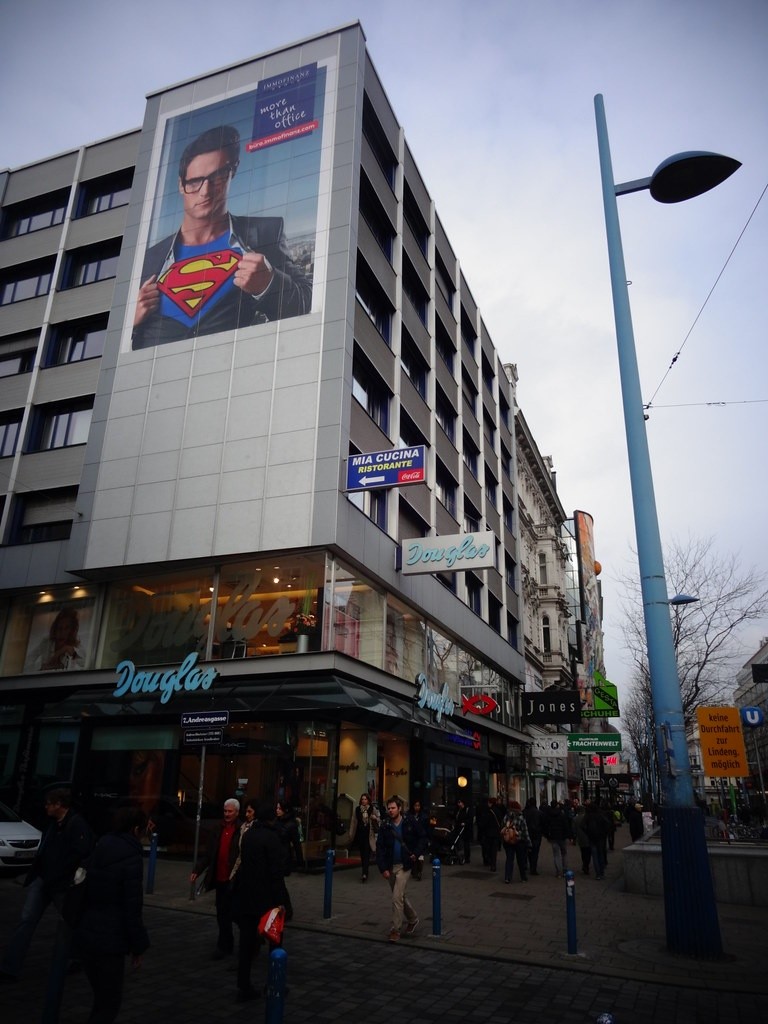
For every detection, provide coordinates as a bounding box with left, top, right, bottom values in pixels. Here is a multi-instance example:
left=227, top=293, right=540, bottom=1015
left=371, top=819, right=379, bottom=833
left=401, top=847, right=419, bottom=871
left=257, top=908, right=285, bottom=944
left=499, top=826, right=504, bottom=837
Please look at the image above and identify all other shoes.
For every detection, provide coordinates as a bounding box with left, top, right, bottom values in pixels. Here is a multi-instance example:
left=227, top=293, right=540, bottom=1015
left=582, top=866, right=590, bottom=877
left=556, top=874, right=562, bottom=880
left=490, top=864, right=497, bottom=872
left=406, top=921, right=419, bottom=934
left=521, top=880, right=528, bottom=883
left=389, top=929, right=401, bottom=941
left=1, top=973, right=17, bottom=984
left=601, top=873, right=604, bottom=879
left=211, top=949, right=233, bottom=961
left=505, top=879, right=511, bottom=884
left=595, top=873, right=601, bottom=881
left=360, top=875, right=367, bottom=883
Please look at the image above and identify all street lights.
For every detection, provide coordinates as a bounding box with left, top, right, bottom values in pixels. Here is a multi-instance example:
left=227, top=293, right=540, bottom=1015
left=589, top=87, right=745, bottom=964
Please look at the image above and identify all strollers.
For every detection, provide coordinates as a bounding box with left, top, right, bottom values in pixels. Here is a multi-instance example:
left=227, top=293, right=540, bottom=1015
left=431, top=824, right=467, bottom=865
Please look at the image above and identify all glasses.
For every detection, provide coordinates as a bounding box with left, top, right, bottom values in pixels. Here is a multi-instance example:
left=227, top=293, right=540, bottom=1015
left=457, top=801, right=461, bottom=804
left=182, top=161, right=235, bottom=194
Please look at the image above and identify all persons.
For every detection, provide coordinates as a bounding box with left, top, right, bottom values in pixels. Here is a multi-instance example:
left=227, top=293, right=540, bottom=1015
left=28, top=607, right=87, bottom=670
left=131, top=126, right=312, bottom=351
left=375, top=797, right=427, bottom=941
left=703, top=814, right=706, bottom=825
left=0, top=788, right=151, bottom=1024
left=349, top=793, right=644, bottom=884
left=191, top=798, right=305, bottom=1002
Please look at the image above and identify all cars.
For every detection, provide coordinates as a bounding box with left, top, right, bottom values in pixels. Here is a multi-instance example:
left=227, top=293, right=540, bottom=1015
left=0, top=800, right=44, bottom=869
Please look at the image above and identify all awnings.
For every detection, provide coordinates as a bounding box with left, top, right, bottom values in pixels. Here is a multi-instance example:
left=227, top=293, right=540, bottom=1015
left=151, top=675, right=479, bottom=843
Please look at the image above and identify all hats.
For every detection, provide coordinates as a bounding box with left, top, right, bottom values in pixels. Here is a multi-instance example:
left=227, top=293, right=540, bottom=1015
left=509, top=801, right=521, bottom=813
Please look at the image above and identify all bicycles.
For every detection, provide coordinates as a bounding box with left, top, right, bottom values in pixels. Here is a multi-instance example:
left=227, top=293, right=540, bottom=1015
left=712, top=819, right=761, bottom=840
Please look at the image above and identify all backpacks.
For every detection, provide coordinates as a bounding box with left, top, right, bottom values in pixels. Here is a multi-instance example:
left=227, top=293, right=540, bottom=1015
left=503, top=817, right=520, bottom=845
